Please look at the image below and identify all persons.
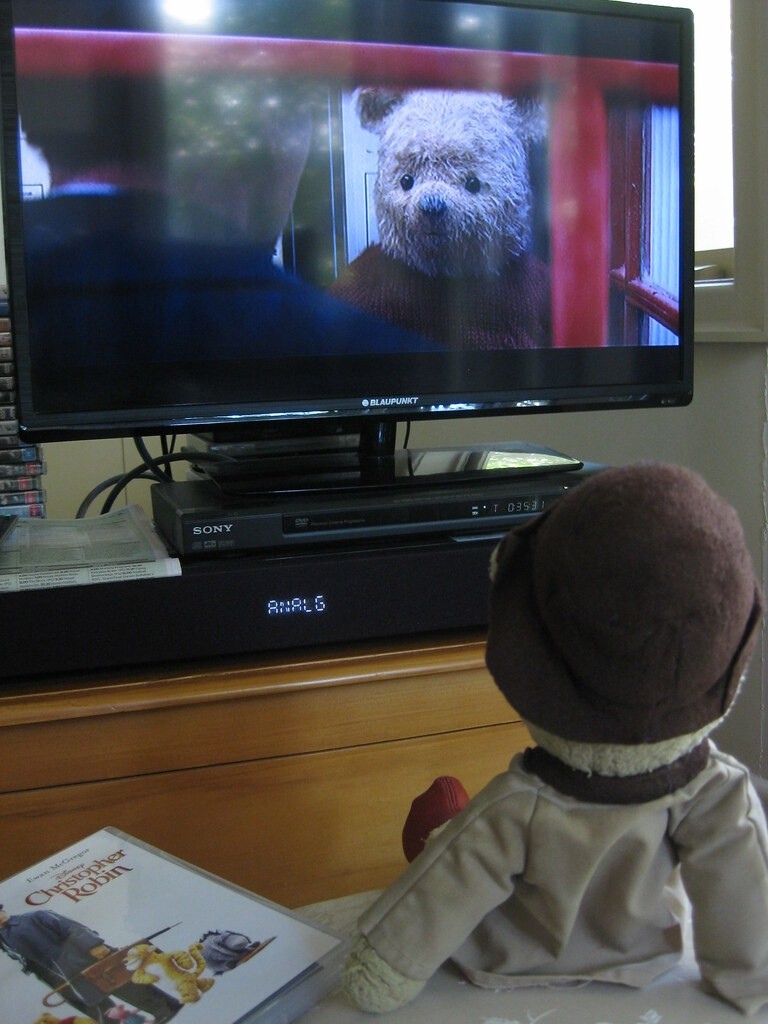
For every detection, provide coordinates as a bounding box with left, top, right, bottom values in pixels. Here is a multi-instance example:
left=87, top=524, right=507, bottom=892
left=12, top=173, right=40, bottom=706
left=20, top=62, right=448, bottom=354
left=0, top=901, right=185, bottom=1024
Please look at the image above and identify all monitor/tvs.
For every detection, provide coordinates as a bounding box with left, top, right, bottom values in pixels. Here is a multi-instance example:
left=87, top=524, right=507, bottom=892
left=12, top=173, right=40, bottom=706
left=0, top=0, right=696, bottom=496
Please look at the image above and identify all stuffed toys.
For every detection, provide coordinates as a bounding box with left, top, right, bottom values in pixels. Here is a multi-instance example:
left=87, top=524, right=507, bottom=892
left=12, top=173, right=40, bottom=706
left=348, top=82, right=560, bottom=362
left=346, top=459, right=768, bottom=1015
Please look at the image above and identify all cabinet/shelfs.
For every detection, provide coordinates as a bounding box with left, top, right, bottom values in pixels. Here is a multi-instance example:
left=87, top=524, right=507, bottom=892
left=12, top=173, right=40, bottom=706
left=0, top=627, right=540, bottom=914
